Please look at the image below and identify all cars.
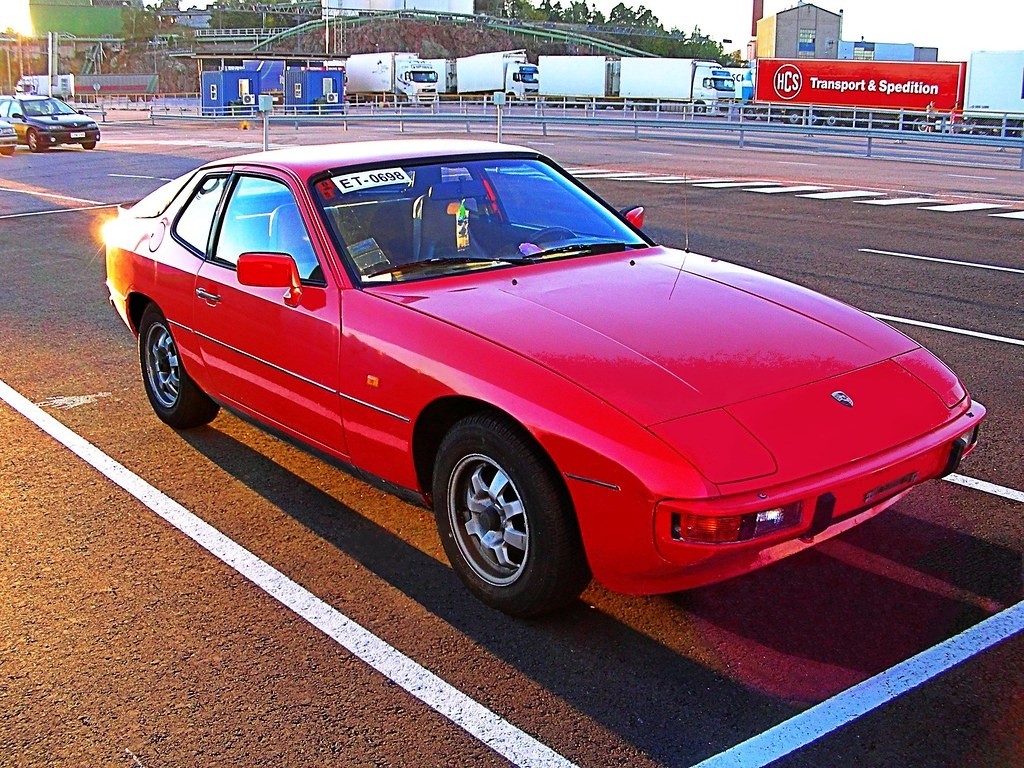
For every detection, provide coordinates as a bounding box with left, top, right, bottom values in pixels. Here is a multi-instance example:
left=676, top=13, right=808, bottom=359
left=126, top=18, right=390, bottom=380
left=0, top=114, right=18, bottom=155
left=103, top=137, right=988, bottom=614
left=0, top=95, right=100, bottom=153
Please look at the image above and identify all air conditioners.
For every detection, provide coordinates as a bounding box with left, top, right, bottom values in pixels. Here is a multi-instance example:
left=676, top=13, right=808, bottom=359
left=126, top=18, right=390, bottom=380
left=326, top=93, right=338, bottom=103
left=243, top=95, right=256, bottom=105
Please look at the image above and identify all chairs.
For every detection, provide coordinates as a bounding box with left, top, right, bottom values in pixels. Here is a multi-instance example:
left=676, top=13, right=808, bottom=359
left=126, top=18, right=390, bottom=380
left=410, top=194, right=487, bottom=258
left=266, top=202, right=318, bottom=269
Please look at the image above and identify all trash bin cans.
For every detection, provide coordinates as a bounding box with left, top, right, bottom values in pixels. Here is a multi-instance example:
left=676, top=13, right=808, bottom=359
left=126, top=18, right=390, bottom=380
left=314, top=97, right=328, bottom=114
left=230, top=100, right=242, bottom=116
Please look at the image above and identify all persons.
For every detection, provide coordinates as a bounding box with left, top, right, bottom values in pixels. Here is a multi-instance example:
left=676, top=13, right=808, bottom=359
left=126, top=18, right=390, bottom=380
left=951, top=101, right=963, bottom=134
left=925, top=100, right=936, bottom=131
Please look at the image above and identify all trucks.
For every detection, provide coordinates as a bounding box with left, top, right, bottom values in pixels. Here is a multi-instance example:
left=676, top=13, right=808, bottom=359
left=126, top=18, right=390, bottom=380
left=620, top=57, right=736, bottom=117
left=423, top=59, right=456, bottom=96
left=456, top=49, right=539, bottom=108
left=16, top=74, right=75, bottom=98
left=346, top=52, right=439, bottom=109
left=538, top=55, right=623, bottom=109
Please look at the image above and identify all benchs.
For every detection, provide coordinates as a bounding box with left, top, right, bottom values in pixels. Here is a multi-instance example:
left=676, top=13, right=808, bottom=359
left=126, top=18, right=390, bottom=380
left=237, top=198, right=412, bottom=258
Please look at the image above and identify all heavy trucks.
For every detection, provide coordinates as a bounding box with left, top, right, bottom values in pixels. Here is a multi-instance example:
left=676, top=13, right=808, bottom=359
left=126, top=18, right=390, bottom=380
left=739, top=58, right=966, bottom=135
left=69, top=74, right=159, bottom=103
left=963, top=49, right=1024, bottom=139
